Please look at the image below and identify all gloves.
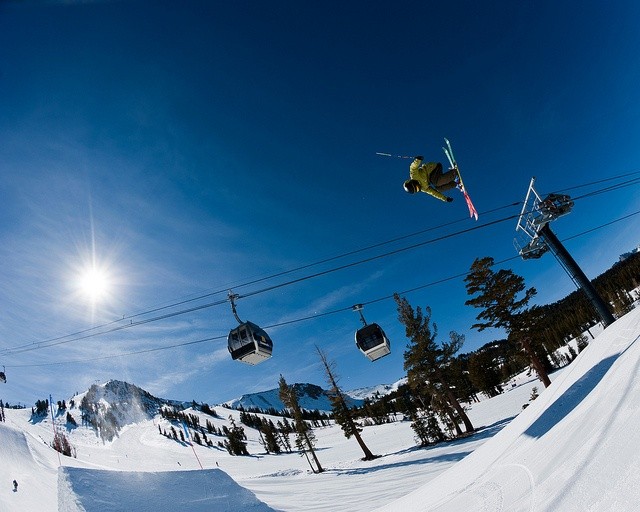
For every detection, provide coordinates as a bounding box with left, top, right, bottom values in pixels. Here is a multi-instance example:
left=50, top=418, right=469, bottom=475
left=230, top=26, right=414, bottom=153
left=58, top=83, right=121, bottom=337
left=446, top=197, right=453, bottom=203
left=416, top=155, right=425, bottom=161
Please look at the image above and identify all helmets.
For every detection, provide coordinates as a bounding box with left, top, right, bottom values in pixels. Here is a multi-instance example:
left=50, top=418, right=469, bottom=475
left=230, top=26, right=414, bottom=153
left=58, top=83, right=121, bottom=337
left=403, top=179, right=421, bottom=193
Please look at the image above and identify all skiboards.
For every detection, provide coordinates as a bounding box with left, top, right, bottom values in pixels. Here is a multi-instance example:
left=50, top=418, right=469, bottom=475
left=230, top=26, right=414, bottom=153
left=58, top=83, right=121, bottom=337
left=440, top=137, right=480, bottom=221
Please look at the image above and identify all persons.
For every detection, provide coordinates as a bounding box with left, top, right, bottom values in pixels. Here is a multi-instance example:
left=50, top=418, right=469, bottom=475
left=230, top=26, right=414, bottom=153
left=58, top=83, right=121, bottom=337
left=13, top=479, right=20, bottom=493
left=403, top=156, right=462, bottom=202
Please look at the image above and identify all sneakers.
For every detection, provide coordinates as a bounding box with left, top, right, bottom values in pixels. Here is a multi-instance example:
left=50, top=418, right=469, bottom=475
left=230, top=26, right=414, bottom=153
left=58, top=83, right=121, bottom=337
left=453, top=169, right=463, bottom=191
